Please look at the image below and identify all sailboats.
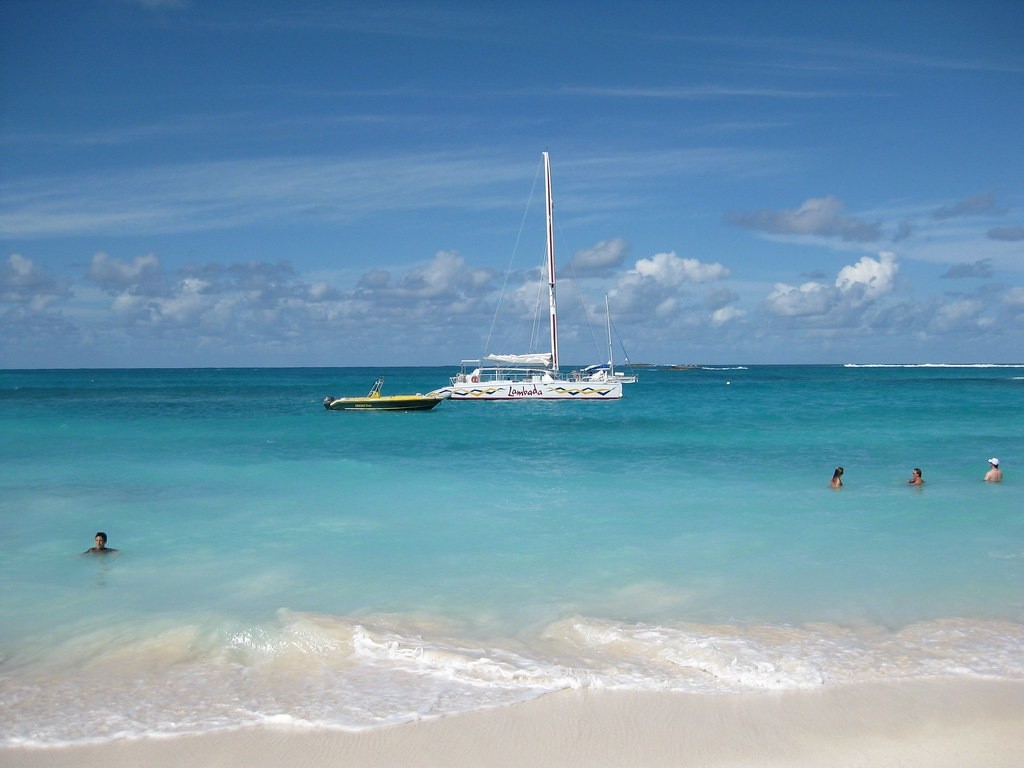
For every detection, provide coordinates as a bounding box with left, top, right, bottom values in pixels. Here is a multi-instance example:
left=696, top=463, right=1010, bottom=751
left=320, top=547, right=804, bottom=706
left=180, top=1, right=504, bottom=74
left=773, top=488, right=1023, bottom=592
left=580, top=291, right=639, bottom=384
left=425, top=149, right=624, bottom=401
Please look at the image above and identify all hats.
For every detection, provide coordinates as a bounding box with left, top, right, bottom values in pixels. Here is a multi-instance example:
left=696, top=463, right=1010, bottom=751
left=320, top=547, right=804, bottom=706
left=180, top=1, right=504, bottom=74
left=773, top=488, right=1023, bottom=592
left=988, top=458, right=999, bottom=465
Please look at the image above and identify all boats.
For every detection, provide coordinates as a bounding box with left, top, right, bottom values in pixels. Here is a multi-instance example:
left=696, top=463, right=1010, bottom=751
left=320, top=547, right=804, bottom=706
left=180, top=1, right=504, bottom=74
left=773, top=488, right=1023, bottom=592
left=671, top=364, right=702, bottom=369
left=732, top=367, right=748, bottom=369
left=323, top=376, right=460, bottom=411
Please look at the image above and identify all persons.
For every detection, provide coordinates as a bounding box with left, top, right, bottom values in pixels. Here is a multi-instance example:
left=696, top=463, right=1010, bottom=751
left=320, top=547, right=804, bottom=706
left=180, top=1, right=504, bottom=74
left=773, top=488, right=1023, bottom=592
left=913, top=468, right=923, bottom=484
left=86, top=532, right=117, bottom=553
left=832, top=467, right=844, bottom=485
left=983, top=458, right=1002, bottom=481
